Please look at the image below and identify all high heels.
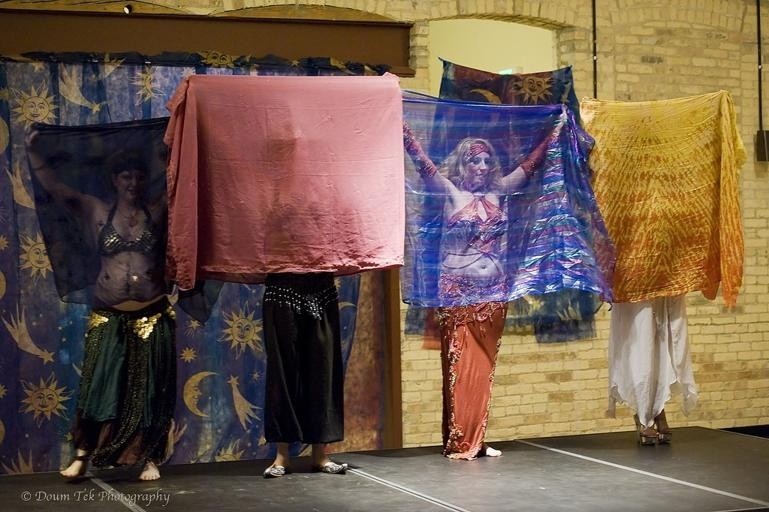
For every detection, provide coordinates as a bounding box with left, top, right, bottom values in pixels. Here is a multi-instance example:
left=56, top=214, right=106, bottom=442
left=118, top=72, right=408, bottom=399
left=633, top=410, right=672, bottom=445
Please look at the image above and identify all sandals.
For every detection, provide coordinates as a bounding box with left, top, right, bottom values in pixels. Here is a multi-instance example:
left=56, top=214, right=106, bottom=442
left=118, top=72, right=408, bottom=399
left=314, top=461, right=349, bottom=474
left=264, top=465, right=291, bottom=476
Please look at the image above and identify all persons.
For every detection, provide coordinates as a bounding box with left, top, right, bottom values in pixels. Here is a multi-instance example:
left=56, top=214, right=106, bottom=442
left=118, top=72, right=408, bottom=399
left=24, top=72, right=732, bottom=483
